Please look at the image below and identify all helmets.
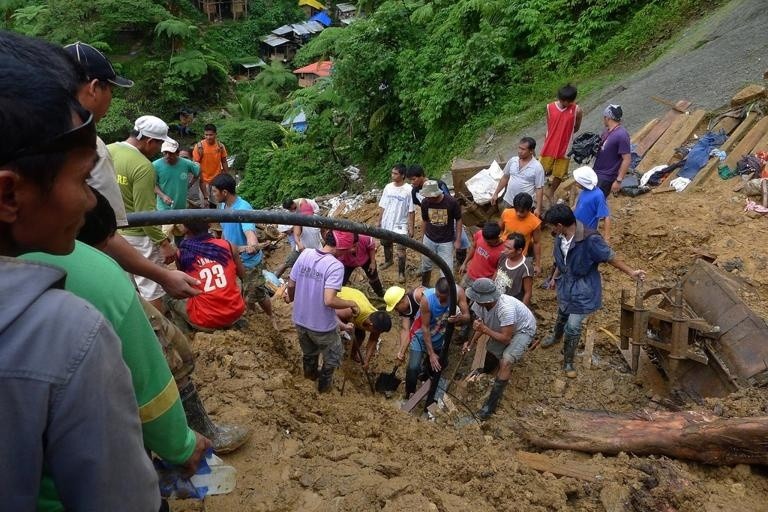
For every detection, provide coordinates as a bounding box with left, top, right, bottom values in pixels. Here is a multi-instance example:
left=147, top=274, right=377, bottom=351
left=383, top=286, right=406, bottom=312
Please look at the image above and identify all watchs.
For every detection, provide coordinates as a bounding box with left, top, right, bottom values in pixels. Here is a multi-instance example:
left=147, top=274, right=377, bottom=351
left=617, top=176, right=624, bottom=183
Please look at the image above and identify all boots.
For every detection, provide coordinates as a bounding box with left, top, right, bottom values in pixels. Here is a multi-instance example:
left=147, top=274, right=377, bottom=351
left=562, top=331, right=580, bottom=379
left=405, top=367, right=416, bottom=398
left=302, top=354, right=320, bottom=381
left=477, top=350, right=500, bottom=375
left=369, top=277, right=386, bottom=297
left=421, top=271, right=431, bottom=287
left=452, top=324, right=470, bottom=345
left=398, top=255, right=407, bottom=283
left=476, top=377, right=509, bottom=418
left=541, top=307, right=570, bottom=348
left=379, top=246, right=394, bottom=270
left=181, top=378, right=254, bottom=455
left=318, top=365, right=335, bottom=393
left=351, top=338, right=361, bottom=362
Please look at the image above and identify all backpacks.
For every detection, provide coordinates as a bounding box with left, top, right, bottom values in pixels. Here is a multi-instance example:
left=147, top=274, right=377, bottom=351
left=569, top=130, right=603, bottom=164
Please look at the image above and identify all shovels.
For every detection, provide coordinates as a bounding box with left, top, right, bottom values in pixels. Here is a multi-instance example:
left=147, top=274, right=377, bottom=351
left=374, top=333, right=411, bottom=396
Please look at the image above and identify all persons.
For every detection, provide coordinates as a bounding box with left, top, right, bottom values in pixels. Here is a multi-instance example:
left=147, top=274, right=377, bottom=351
left=558, top=165, right=612, bottom=243
left=339, top=231, right=385, bottom=300
left=591, top=105, right=630, bottom=199
left=539, top=84, right=582, bottom=200
left=378, top=163, right=416, bottom=283
left=174, top=205, right=246, bottom=332
left=417, top=180, right=463, bottom=285
left=207, top=174, right=278, bottom=332
left=407, top=164, right=470, bottom=264
left=459, top=222, right=505, bottom=301
left=492, top=233, right=533, bottom=304
left=464, top=278, right=538, bottom=421
left=180, top=147, right=204, bottom=209
left=82, top=180, right=119, bottom=253
left=1, top=27, right=160, bottom=512
left=282, top=227, right=359, bottom=393
left=403, top=276, right=470, bottom=418
left=61, top=39, right=249, bottom=453
left=193, top=123, right=228, bottom=194
left=278, top=225, right=297, bottom=250
left=498, top=192, right=542, bottom=278
left=541, top=204, right=646, bottom=377
left=16, top=237, right=214, bottom=484
left=284, top=198, right=319, bottom=216
left=490, top=138, right=544, bottom=217
left=276, top=203, right=324, bottom=279
left=335, top=285, right=392, bottom=372
left=384, top=283, right=429, bottom=362
left=153, top=140, right=200, bottom=211
left=105, top=115, right=178, bottom=356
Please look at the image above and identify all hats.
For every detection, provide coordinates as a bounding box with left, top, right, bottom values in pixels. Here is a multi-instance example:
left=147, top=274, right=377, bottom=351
left=418, top=180, right=443, bottom=198
left=134, top=115, right=171, bottom=141
left=464, top=277, right=501, bottom=303
left=63, top=40, right=135, bottom=88
left=160, top=139, right=180, bottom=153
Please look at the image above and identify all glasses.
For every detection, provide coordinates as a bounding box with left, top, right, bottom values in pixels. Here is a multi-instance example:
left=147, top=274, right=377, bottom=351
left=179, top=155, right=190, bottom=159
left=0, top=110, right=104, bottom=167
